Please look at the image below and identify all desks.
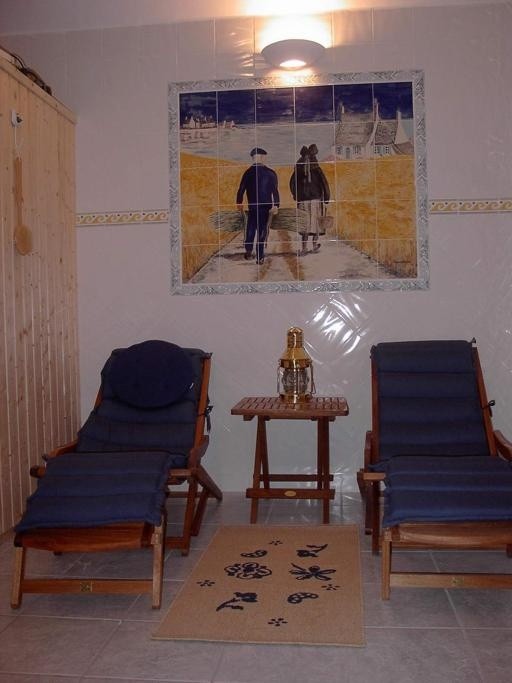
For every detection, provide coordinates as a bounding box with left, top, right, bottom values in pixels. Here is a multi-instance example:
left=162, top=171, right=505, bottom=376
left=229, top=394, right=350, bottom=525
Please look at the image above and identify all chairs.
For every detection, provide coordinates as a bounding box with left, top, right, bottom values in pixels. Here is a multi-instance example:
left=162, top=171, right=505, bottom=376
left=355, top=336, right=512, bottom=601
left=5, top=339, right=224, bottom=614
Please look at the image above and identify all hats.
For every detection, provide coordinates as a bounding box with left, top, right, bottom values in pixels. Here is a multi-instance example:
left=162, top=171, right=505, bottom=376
left=300, top=144, right=318, bottom=155
left=250, top=148, right=267, bottom=156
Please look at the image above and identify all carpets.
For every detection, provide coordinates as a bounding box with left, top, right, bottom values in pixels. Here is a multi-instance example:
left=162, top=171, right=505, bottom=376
left=148, top=522, right=367, bottom=648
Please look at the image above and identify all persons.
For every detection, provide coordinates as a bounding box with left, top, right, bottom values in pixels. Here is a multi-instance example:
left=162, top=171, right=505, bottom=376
left=237, top=148, right=280, bottom=265
left=289, top=144, right=330, bottom=256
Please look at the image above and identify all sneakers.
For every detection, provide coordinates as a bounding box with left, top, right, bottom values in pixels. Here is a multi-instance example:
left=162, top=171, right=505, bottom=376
left=244, top=250, right=253, bottom=260
left=256, top=255, right=264, bottom=265
left=313, top=243, right=321, bottom=252
left=301, top=248, right=312, bottom=256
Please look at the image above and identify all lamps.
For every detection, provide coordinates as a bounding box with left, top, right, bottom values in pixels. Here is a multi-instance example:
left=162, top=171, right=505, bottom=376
left=272, top=323, right=319, bottom=406
left=259, top=38, right=327, bottom=69
